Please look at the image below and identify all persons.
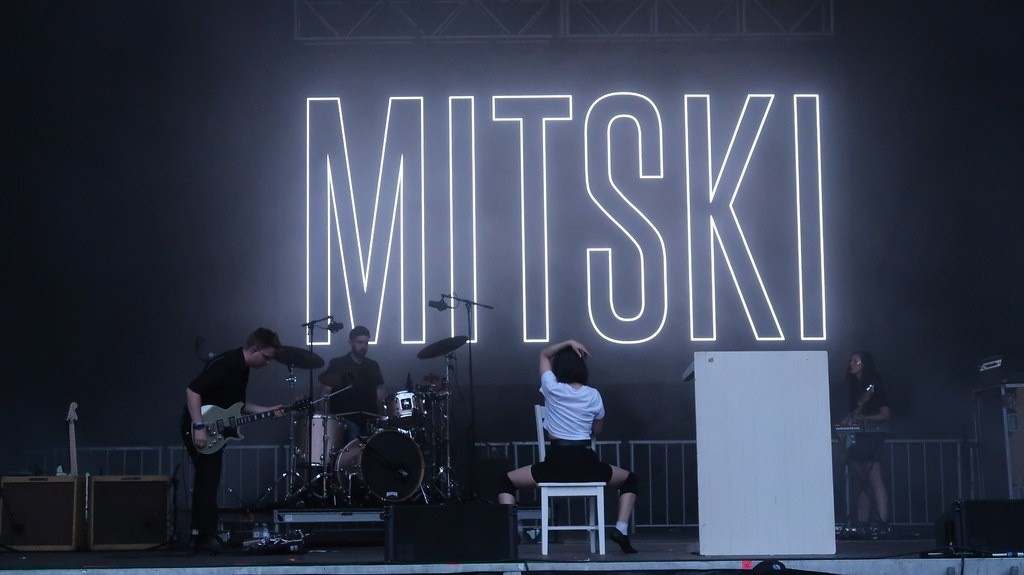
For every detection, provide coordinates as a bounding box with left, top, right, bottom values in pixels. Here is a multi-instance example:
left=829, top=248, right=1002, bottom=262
left=180, top=326, right=284, bottom=554
left=497, top=339, right=640, bottom=553
left=318, top=326, right=386, bottom=440
left=834, top=350, right=891, bottom=537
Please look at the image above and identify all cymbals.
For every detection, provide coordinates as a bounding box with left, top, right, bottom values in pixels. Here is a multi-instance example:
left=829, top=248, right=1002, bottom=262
left=273, top=345, right=326, bottom=370
left=417, top=335, right=469, bottom=360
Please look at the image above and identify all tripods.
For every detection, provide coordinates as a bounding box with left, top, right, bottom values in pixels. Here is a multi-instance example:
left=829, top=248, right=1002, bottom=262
left=147, top=465, right=194, bottom=551
left=245, top=315, right=351, bottom=516
left=408, top=353, right=495, bottom=504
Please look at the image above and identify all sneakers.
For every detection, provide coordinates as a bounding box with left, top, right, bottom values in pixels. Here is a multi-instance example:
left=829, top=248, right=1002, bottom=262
left=609, top=527, right=638, bottom=553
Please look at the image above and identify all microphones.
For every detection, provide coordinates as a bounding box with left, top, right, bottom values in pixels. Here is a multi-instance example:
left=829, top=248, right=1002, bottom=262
left=328, top=323, right=343, bottom=330
left=406, top=373, right=412, bottom=390
left=397, top=469, right=409, bottom=480
left=428, top=300, right=452, bottom=310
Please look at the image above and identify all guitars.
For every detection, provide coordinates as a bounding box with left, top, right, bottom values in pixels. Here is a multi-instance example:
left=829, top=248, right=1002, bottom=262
left=190, top=394, right=313, bottom=455
left=843, top=383, right=876, bottom=454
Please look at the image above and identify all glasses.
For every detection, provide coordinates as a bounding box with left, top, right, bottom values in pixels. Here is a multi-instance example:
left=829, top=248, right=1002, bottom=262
left=255, top=347, right=274, bottom=364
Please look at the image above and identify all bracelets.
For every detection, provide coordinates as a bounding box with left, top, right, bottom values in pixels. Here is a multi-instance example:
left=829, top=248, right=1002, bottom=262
left=193, top=423, right=205, bottom=430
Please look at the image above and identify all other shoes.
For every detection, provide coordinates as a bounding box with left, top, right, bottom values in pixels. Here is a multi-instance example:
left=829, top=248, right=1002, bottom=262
left=848, top=527, right=892, bottom=538
left=186, top=535, right=226, bottom=552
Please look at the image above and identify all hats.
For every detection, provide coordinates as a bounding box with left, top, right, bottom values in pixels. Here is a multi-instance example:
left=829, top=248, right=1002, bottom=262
left=349, top=326, right=370, bottom=339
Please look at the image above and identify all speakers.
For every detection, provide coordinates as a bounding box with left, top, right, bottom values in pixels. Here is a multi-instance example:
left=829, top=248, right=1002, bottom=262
left=0, top=476, right=87, bottom=551
left=936, top=498, right=1024, bottom=559
left=380, top=504, right=521, bottom=564
left=89, top=474, right=173, bottom=550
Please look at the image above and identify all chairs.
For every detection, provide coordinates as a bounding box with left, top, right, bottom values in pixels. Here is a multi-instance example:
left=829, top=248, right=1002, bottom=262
left=535, top=404, right=608, bottom=559
left=961, top=499, right=1024, bottom=546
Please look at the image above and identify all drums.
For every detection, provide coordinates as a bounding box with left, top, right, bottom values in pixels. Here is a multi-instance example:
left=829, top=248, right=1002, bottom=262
left=386, top=388, right=426, bottom=432
left=293, top=413, right=349, bottom=470
left=334, top=429, right=425, bottom=503
